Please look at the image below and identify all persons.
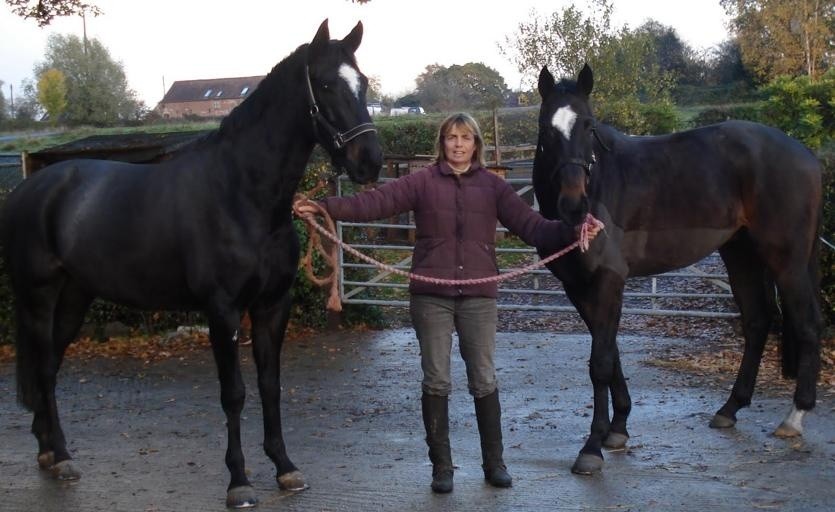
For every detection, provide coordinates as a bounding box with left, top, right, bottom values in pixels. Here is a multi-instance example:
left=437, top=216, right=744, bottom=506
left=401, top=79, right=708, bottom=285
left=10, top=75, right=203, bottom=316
left=292, top=112, right=607, bottom=494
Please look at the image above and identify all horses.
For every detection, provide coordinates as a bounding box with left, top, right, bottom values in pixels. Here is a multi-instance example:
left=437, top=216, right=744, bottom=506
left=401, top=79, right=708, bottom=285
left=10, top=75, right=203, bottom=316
left=530, top=61, right=826, bottom=477
left=0, top=18, right=385, bottom=509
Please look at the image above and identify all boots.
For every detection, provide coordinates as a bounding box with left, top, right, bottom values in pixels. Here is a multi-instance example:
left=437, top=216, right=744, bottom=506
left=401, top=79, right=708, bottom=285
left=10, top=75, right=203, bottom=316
left=421, top=392, right=454, bottom=493
left=474, top=389, right=512, bottom=488
